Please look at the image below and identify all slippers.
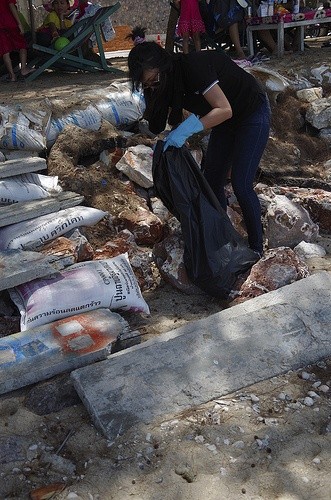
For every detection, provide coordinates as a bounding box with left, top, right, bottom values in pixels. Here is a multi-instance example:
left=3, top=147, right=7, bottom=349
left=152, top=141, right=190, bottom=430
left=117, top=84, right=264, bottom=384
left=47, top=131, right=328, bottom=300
left=256, top=51, right=268, bottom=60
left=247, top=54, right=262, bottom=65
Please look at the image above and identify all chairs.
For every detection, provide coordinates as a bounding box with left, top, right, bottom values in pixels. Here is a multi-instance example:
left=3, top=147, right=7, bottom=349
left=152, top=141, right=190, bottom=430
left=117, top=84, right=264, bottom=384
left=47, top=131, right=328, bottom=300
left=25, top=2, right=121, bottom=82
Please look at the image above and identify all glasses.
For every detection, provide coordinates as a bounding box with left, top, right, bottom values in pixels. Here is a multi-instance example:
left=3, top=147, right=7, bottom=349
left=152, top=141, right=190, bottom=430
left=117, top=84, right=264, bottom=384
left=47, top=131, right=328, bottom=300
left=141, top=72, right=160, bottom=91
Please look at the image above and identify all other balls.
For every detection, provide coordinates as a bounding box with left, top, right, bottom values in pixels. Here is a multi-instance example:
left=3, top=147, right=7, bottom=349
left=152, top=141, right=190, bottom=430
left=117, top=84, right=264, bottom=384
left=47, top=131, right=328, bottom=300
left=54, top=37, right=70, bottom=51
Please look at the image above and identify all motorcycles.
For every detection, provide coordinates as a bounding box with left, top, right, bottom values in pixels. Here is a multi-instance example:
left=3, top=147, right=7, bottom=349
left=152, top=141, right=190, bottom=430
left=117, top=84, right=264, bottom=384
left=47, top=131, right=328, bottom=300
left=173, top=24, right=225, bottom=53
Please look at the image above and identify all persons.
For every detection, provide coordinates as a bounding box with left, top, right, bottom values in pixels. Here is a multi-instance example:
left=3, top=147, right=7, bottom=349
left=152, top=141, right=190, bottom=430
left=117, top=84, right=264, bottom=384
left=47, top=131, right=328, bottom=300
left=0, top=0, right=93, bottom=82
left=173, top=0, right=331, bottom=59
left=127, top=26, right=272, bottom=253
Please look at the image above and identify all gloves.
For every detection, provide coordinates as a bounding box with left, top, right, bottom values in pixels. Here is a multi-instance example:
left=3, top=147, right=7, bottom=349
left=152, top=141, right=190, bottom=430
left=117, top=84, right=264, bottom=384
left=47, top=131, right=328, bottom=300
left=163, top=113, right=204, bottom=152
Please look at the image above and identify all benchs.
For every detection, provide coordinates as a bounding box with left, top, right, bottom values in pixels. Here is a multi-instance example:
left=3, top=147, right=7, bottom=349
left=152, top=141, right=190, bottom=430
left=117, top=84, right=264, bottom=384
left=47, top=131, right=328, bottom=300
left=246, top=7, right=331, bottom=56
